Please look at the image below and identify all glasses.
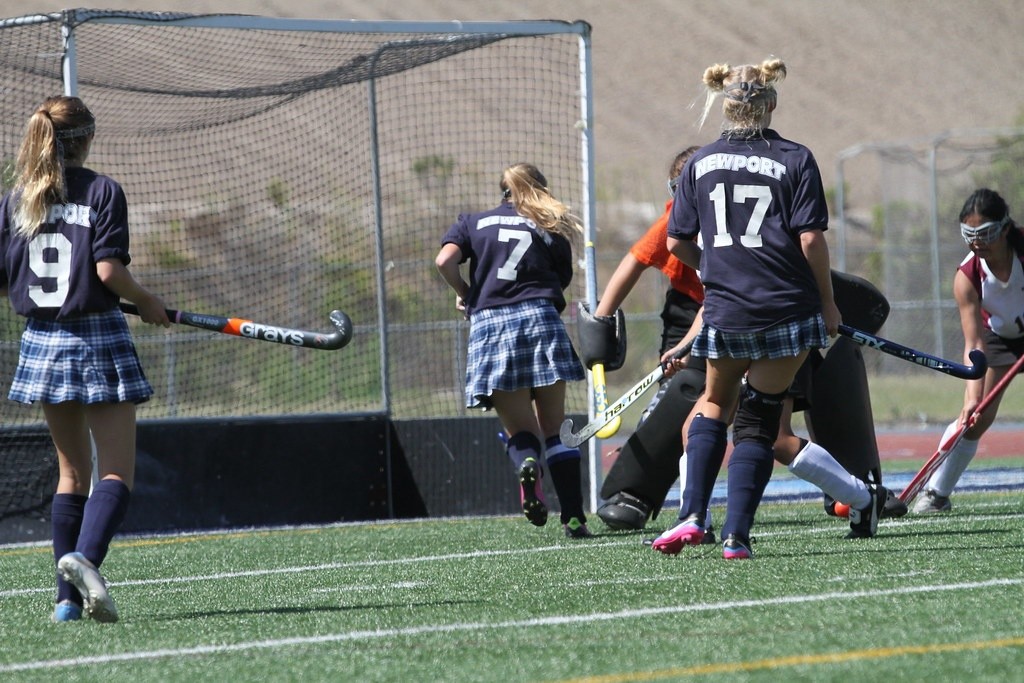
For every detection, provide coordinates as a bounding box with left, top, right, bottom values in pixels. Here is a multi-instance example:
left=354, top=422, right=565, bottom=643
left=960, top=211, right=1009, bottom=245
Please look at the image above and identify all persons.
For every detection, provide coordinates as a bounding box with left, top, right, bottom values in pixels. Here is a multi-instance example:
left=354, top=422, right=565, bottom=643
left=435, top=161, right=596, bottom=539
left=910, top=187, right=1024, bottom=516
left=651, top=56, right=844, bottom=558
left=642, top=296, right=890, bottom=543
left=593, top=145, right=706, bottom=531
left=0, top=94, right=171, bottom=625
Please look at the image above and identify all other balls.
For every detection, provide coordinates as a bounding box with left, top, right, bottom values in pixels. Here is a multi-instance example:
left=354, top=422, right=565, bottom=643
left=834, top=498, right=851, bottom=516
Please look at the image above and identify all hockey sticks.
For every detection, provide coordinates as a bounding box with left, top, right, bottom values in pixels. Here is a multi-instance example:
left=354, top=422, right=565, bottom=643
left=837, top=324, right=989, bottom=380
left=558, top=336, right=695, bottom=450
left=583, top=238, right=624, bottom=441
left=899, top=352, right=1023, bottom=506
left=118, top=301, right=352, bottom=351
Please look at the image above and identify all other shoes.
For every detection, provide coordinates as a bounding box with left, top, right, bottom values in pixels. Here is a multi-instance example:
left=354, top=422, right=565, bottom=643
left=597, top=491, right=652, bottom=529
left=824, top=489, right=908, bottom=518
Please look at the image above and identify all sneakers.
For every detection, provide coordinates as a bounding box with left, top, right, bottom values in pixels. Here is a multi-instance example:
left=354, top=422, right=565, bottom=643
left=51, top=599, right=83, bottom=622
left=651, top=512, right=705, bottom=553
left=57, top=552, right=119, bottom=623
left=722, top=533, right=752, bottom=559
left=642, top=524, right=716, bottom=544
left=519, top=457, right=548, bottom=526
left=912, top=488, right=951, bottom=513
left=563, top=517, right=593, bottom=538
left=844, top=484, right=887, bottom=539
